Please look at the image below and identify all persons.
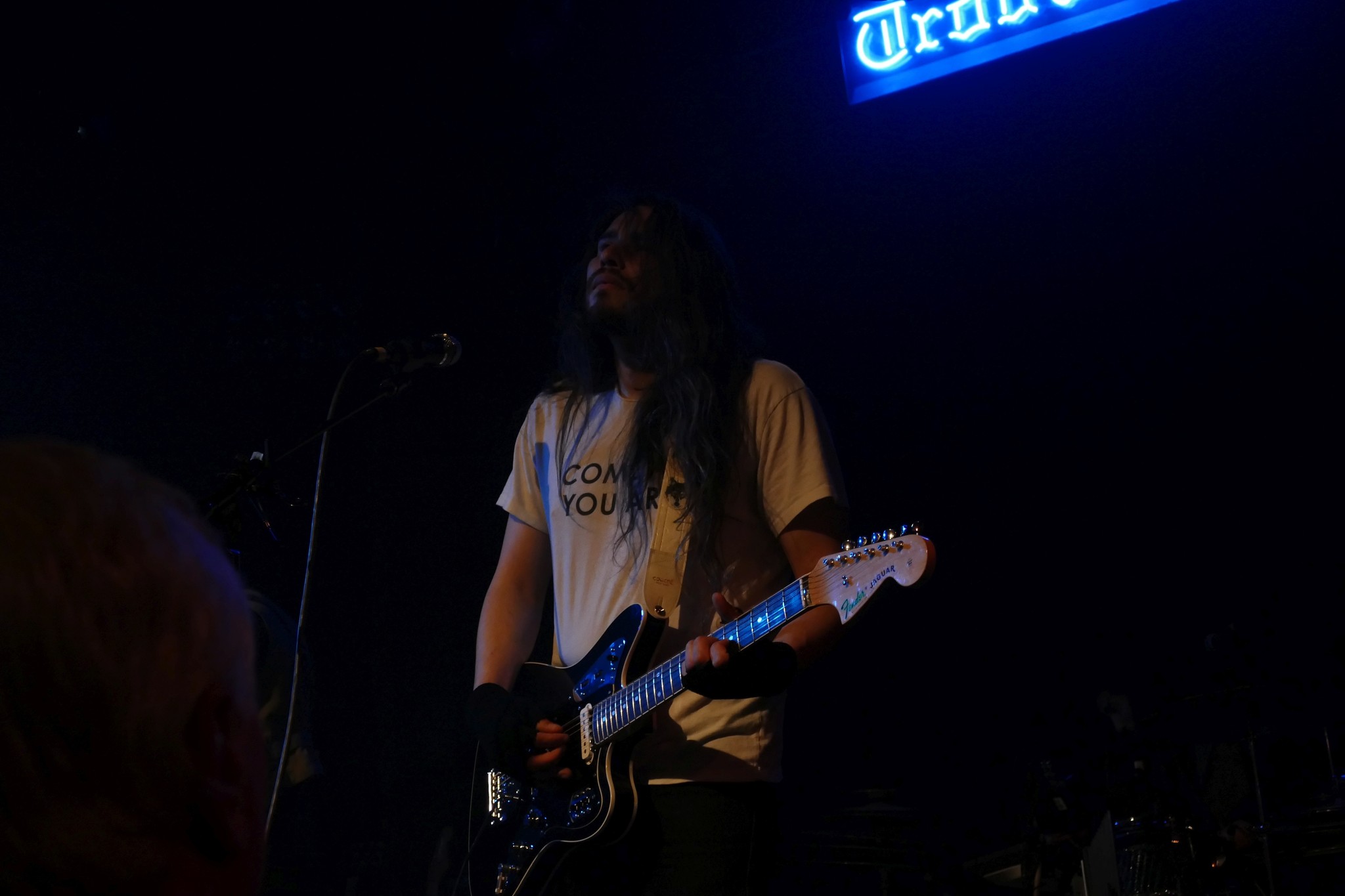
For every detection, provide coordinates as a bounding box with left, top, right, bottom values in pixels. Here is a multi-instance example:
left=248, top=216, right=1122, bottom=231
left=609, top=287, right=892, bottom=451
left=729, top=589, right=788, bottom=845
left=464, top=200, right=846, bottom=896
left=0, top=440, right=269, bottom=896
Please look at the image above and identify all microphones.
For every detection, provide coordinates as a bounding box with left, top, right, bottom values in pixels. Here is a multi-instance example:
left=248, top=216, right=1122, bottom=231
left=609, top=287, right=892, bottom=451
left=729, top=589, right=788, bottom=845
left=358, top=333, right=461, bottom=369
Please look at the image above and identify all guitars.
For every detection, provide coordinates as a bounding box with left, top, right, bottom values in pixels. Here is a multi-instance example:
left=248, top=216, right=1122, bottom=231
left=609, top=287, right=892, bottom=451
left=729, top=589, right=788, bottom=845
left=466, top=518, right=937, bottom=896
